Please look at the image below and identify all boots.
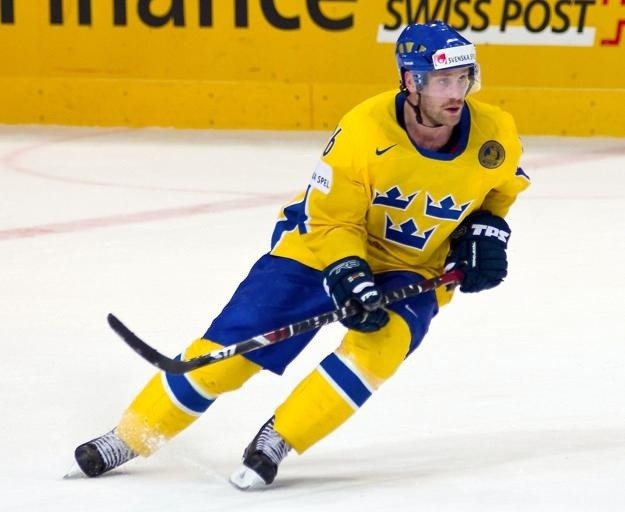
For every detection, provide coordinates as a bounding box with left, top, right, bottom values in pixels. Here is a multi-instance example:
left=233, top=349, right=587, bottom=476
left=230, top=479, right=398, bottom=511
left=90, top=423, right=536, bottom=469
left=243, top=414, right=294, bottom=484
left=73, top=426, right=138, bottom=477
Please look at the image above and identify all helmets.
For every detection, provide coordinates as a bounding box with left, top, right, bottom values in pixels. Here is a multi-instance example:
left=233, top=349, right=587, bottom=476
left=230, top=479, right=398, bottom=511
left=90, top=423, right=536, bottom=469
left=394, top=21, right=476, bottom=92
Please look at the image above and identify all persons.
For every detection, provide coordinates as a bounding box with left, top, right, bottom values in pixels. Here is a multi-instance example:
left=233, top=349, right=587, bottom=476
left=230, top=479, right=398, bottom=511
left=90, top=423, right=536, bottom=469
left=62, top=20, right=534, bottom=491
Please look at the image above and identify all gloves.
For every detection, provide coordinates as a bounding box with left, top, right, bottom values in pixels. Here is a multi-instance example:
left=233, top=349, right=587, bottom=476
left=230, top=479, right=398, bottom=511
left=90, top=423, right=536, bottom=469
left=322, top=256, right=391, bottom=334
left=447, top=209, right=512, bottom=292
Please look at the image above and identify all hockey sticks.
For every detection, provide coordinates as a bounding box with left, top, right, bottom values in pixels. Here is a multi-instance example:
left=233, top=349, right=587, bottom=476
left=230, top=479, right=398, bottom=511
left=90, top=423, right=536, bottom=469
left=107, top=270, right=464, bottom=375
